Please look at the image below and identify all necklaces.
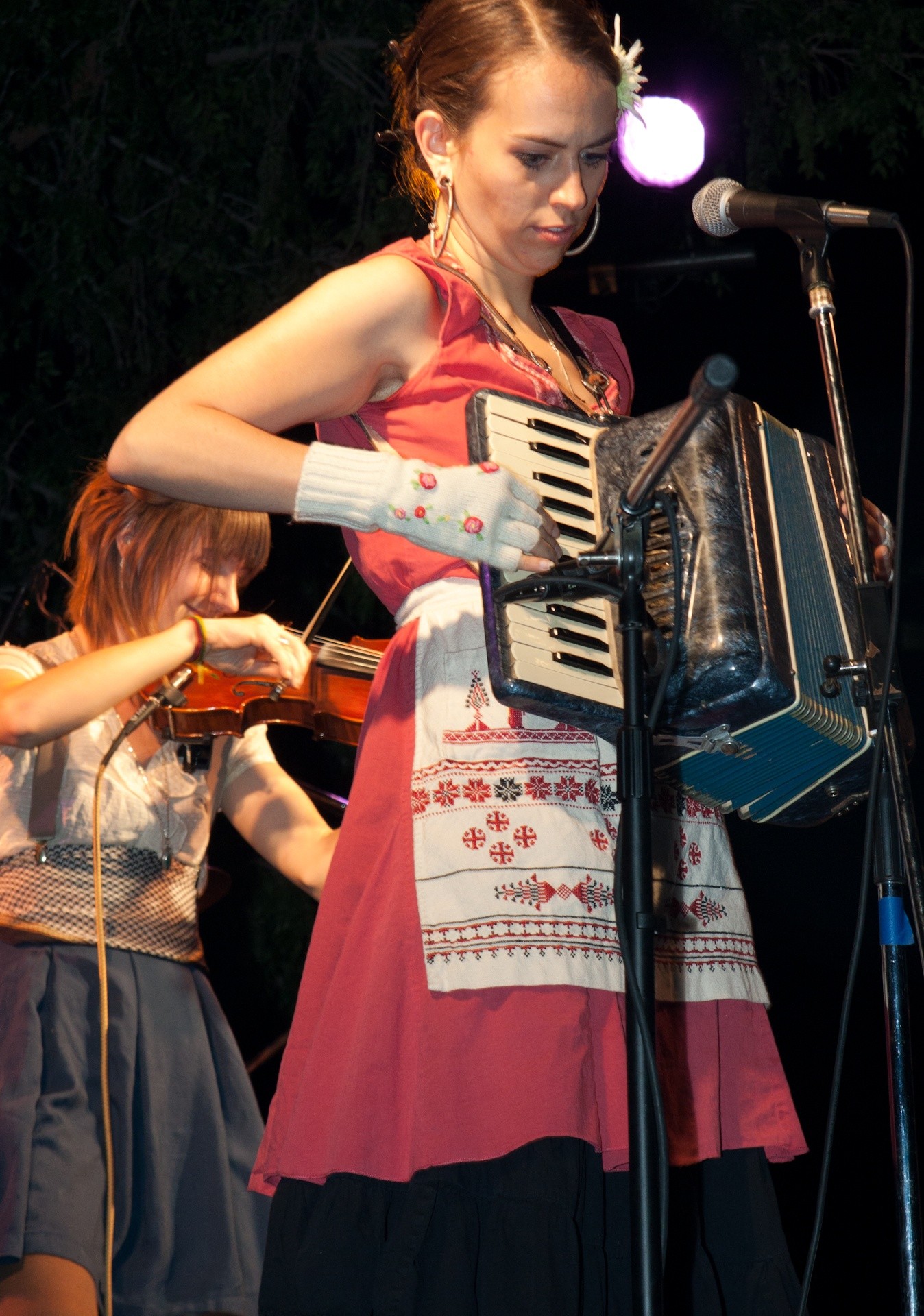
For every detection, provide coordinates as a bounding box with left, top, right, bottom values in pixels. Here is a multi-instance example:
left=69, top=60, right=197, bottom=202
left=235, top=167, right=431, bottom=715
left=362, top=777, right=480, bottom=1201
left=441, top=248, right=582, bottom=401
left=115, top=708, right=175, bottom=869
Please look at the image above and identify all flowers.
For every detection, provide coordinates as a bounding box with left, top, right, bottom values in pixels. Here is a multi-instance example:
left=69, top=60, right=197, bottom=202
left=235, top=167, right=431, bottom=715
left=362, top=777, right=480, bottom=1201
left=612, top=13, right=650, bottom=136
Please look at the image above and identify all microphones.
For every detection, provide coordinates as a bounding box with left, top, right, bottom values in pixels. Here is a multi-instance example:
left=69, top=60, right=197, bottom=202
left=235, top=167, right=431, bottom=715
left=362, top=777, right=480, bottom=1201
left=119, top=667, right=191, bottom=736
left=692, top=177, right=899, bottom=238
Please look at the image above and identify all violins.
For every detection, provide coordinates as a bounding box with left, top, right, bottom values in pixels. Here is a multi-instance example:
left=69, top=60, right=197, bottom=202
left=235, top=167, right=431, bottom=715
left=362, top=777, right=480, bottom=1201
left=128, top=609, right=394, bottom=747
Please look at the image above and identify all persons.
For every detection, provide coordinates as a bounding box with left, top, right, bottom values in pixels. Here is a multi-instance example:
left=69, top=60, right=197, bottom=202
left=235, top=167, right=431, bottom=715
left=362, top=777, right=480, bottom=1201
left=0, top=455, right=338, bottom=1316
left=105, top=1, right=894, bottom=1316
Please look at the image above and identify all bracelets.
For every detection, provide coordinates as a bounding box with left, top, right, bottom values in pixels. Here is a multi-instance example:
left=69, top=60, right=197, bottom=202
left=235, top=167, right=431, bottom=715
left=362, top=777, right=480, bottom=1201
left=183, top=615, right=209, bottom=667
